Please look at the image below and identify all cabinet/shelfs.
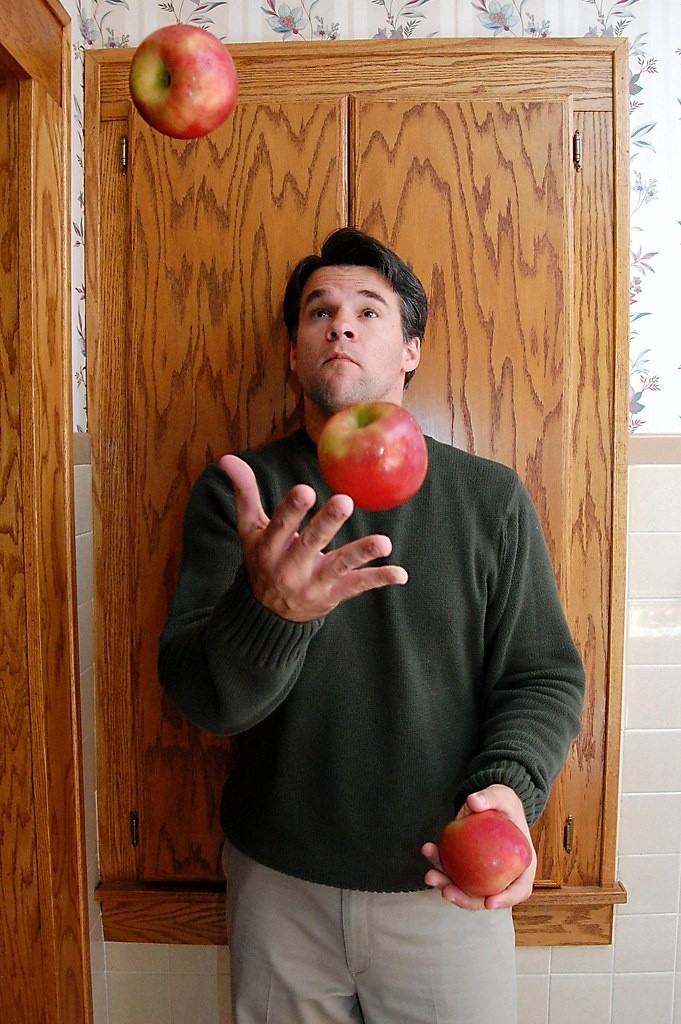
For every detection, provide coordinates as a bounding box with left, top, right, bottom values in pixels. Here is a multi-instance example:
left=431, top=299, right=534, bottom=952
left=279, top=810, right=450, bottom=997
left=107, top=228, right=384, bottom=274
left=82, top=40, right=628, bottom=945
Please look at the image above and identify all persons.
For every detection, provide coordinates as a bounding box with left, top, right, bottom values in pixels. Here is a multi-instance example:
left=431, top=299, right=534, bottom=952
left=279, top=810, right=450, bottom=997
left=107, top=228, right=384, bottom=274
left=157, top=225, right=587, bottom=1024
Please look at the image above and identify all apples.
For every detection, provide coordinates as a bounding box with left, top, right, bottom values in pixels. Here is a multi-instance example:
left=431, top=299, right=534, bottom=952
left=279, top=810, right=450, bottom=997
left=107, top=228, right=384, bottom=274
left=319, top=401, right=428, bottom=510
left=129, top=24, right=238, bottom=140
left=440, top=811, right=532, bottom=898
